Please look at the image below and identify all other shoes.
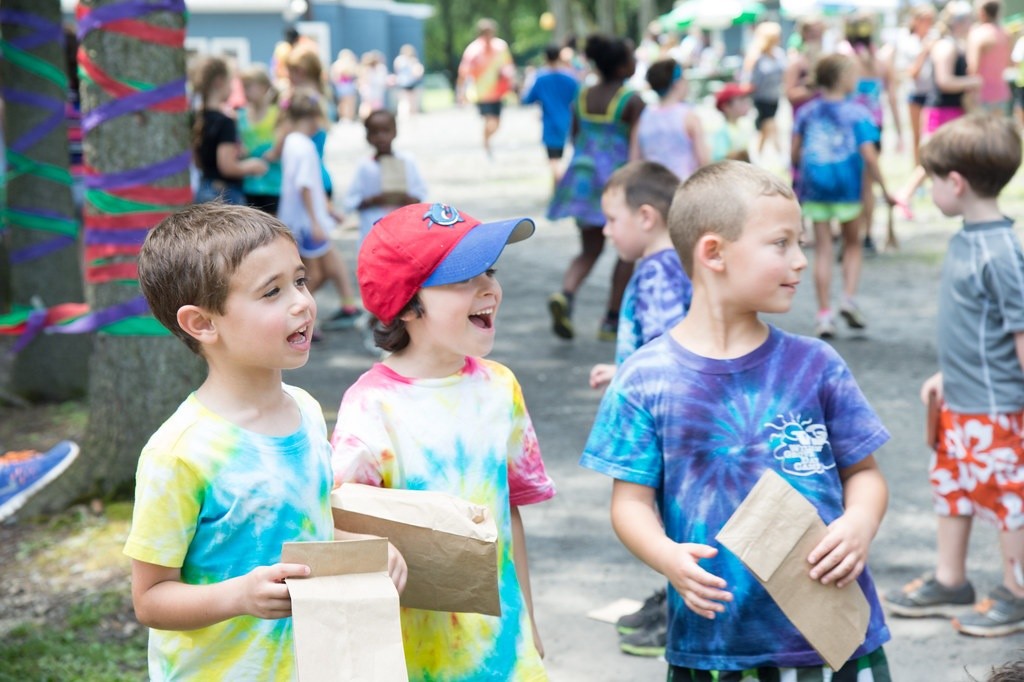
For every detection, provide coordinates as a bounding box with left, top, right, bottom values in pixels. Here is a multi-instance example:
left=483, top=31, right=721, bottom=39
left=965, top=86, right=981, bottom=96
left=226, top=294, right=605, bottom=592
left=817, top=317, right=836, bottom=338
left=840, top=301, right=864, bottom=328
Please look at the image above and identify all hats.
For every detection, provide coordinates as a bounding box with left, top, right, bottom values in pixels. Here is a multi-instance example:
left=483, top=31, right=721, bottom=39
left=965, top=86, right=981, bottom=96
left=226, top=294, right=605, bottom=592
left=717, top=83, right=756, bottom=109
left=357, top=204, right=535, bottom=327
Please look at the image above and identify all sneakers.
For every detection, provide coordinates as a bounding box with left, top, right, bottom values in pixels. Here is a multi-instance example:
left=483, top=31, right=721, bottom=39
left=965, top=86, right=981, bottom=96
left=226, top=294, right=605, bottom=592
left=882, top=569, right=975, bottom=618
left=0, top=440, right=80, bottom=523
left=599, top=319, right=619, bottom=341
left=951, top=584, right=1024, bottom=636
left=547, top=293, right=574, bottom=338
left=620, top=620, right=667, bottom=656
left=616, top=589, right=667, bottom=633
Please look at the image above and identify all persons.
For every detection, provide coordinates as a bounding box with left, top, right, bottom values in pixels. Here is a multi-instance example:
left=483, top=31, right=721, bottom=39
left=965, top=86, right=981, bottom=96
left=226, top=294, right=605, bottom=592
left=518, top=1, right=1024, bottom=344
left=120, top=196, right=410, bottom=681
left=579, top=159, right=893, bottom=682
left=182, top=27, right=430, bottom=339
left=589, top=161, right=692, bottom=656
left=455, top=17, right=520, bottom=154
left=878, top=111, right=1023, bottom=637
left=329, top=202, right=557, bottom=682
